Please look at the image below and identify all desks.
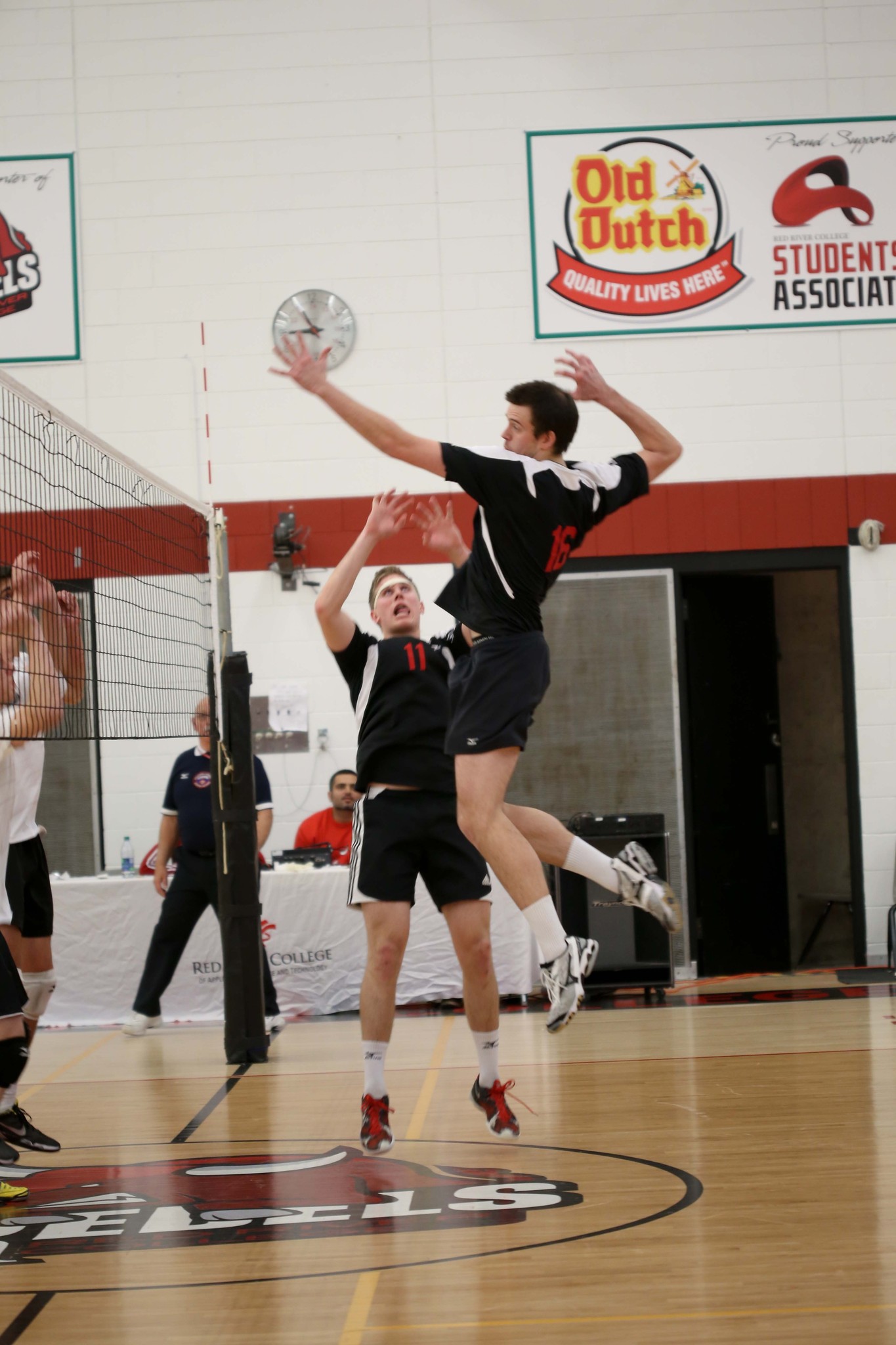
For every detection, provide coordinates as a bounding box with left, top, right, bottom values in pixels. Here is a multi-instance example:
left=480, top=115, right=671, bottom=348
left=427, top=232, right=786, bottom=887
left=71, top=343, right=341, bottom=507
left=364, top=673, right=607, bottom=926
left=18, top=863, right=545, bottom=1026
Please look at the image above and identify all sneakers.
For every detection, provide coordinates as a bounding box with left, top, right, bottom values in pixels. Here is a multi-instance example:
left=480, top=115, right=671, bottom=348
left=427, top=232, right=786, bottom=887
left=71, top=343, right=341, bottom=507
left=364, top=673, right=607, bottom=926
left=123, top=1010, right=162, bottom=1036
left=0, top=1141, right=20, bottom=1165
left=360, top=1094, right=395, bottom=1154
left=0, top=1180, right=29, bottom=1201
left=469, top=1072, right=537, bottom=1141
left=592, top=841, right=682, bottom=933
left=0, top=1099, right=61, bottom=1152
left=539, top=936, right=600, bottom=1035
left=264, top=1014, right=285, bottom=1032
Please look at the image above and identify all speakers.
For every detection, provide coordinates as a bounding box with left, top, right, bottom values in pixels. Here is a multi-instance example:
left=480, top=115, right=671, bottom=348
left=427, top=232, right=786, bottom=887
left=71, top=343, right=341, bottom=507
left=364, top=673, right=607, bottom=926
left=554, top=832, right=675, bottom=991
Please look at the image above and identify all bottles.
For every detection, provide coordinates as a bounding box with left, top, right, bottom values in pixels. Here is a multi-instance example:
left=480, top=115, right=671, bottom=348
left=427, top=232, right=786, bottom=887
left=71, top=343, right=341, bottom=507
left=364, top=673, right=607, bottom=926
left=121, top=836, right=134, bottom=877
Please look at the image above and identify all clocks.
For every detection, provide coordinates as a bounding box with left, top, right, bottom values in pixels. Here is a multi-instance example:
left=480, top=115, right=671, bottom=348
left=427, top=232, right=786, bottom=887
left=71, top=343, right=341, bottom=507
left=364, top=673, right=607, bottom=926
left=273, top=289, right=355, bottom=371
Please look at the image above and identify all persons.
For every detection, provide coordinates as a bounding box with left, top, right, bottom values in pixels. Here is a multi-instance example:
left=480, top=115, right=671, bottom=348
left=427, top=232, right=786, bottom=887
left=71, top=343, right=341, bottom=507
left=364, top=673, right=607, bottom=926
left=266, top=331, right=680, bottom=1034
left=317, top=488, right=523, bottom=1152
left=0, top=552, right=362, bottom=1167
